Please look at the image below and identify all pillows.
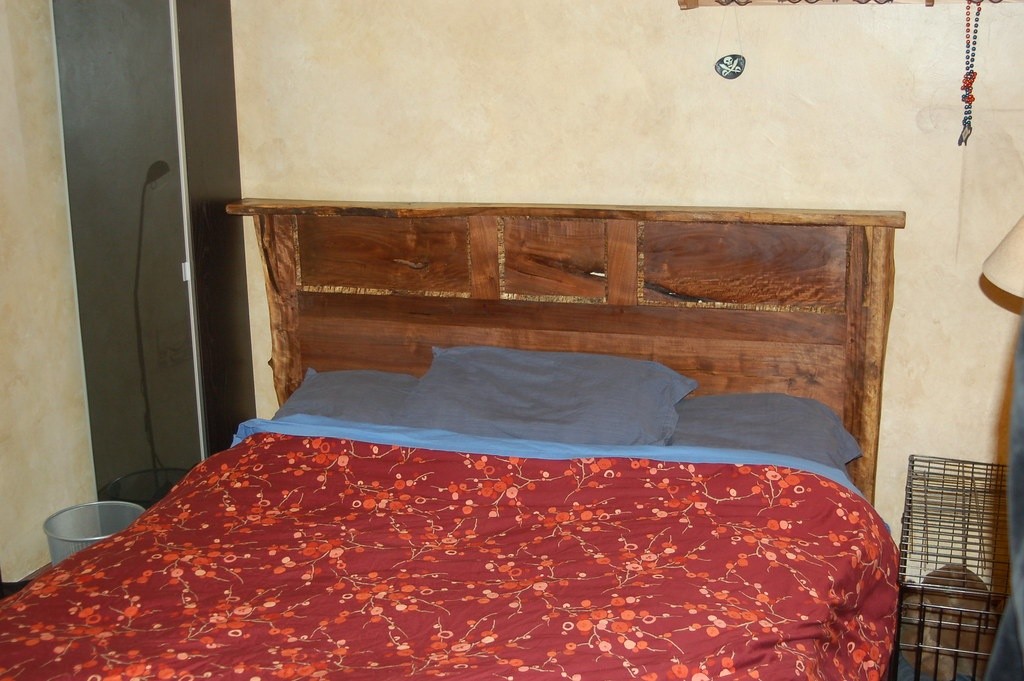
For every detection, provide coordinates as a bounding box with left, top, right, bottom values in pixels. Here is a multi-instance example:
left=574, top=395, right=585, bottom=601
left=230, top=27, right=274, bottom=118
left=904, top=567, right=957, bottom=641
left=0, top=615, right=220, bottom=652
left=391, top=346, right=699, bottom=447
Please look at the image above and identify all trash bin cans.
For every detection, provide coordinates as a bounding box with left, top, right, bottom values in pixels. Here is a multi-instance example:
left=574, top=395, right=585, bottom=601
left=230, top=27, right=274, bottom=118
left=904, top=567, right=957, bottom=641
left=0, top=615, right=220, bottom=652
left=99, top=465, right=191, bottom=512
left=43, top=500, right=144, bottom=570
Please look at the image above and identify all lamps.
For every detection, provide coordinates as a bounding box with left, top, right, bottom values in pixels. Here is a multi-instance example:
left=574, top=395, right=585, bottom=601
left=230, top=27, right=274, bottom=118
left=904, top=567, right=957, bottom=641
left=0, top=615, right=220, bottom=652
left=978, top=214, right=1024, bottom=316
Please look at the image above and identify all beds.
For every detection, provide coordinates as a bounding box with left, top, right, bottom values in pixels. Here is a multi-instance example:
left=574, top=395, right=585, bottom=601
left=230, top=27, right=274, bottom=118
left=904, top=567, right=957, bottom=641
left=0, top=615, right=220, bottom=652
left=0, top=198, right=907, bottom=681
